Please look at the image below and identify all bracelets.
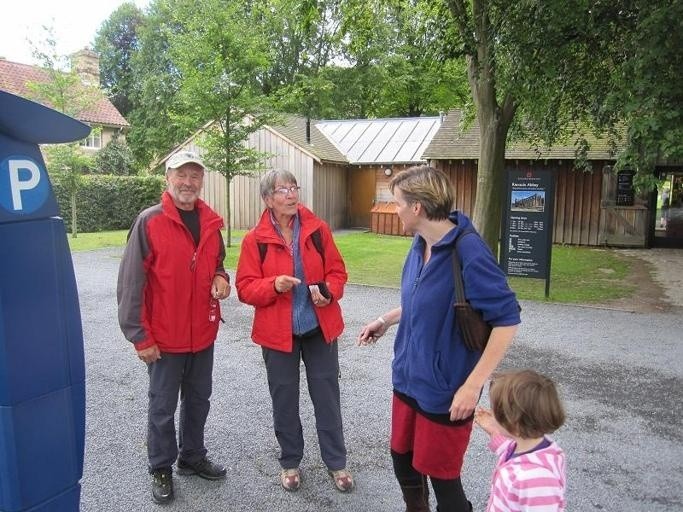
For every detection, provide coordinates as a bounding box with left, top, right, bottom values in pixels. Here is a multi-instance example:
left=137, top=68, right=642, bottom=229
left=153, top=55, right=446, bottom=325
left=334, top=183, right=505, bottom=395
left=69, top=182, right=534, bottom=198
left=378, top=317, right=390, bottom=329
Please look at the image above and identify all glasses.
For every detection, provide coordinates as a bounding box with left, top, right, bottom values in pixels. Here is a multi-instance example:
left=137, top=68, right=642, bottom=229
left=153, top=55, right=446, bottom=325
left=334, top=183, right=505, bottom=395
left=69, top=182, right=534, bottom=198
left=209, top=298, right=225, bottom=323
left=273, top=186, right=300, bottom=194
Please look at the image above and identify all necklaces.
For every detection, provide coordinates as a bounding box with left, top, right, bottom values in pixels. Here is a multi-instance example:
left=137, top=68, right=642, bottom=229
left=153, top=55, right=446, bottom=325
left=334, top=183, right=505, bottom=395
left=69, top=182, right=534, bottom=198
left=277, top=216, right=295, bottom=233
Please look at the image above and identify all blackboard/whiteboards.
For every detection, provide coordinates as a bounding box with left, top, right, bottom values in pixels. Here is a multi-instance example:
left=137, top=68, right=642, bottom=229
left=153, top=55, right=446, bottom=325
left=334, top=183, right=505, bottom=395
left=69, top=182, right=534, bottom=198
left=616, top=170, right=636, bottom=206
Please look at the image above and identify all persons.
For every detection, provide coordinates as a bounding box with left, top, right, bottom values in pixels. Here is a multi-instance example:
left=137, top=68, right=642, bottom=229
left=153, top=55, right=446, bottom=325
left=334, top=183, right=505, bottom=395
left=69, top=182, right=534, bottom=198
left=234, top=168, right=355, bottom=494
left=473, top=369, right=567, bottom=512
left=114, top=151, right=232, bottom=504
left=355, top=165, right=524, bottom=512
left=659, top=187, right=670, bottom=228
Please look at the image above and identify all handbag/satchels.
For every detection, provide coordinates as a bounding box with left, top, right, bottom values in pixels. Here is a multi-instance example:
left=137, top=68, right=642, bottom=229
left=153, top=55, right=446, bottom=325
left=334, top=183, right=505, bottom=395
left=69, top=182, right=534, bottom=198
left=453, top=301, right=522, bottom=352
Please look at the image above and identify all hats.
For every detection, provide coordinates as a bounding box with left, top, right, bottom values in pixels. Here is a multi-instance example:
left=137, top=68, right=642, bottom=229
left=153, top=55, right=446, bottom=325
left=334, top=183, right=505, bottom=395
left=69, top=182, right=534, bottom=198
left=166, top=152, right=205, bottom=172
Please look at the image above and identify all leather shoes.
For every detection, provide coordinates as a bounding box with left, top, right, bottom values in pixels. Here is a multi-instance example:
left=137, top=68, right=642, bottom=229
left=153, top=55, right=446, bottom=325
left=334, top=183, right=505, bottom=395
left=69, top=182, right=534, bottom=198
left=152, top=469, right=173, bottom=503
left=178, top=458, right=226, bottom=480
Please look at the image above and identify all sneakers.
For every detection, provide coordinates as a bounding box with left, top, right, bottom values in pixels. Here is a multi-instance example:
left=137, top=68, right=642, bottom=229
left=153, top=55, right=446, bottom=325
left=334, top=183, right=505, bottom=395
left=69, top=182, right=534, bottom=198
left=282, top=468, right=300, bottom=491
left=328, top=469, right=353, bottom=492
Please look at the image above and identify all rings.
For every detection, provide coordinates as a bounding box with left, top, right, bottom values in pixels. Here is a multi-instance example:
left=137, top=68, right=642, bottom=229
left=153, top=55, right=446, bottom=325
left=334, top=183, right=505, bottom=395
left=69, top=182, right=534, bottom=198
left=314, top=300, right=319, bottom=305
